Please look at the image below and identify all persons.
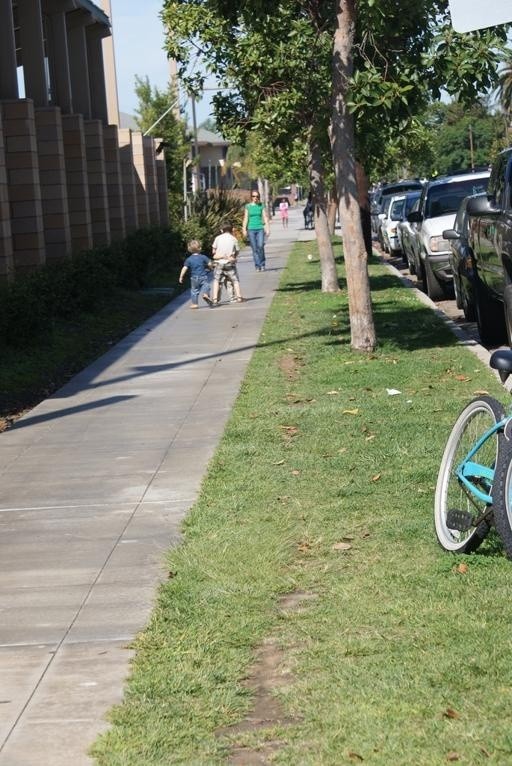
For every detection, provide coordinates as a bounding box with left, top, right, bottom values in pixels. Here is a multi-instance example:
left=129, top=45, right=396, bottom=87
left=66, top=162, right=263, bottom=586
left=211, top=254, right=238, bottom=303
left=209, top=220, right=244, bottom=305
left=279, top=198, right=289, bottom=231
left=240, top=188, right=270, bottom=272
left=302, top=190, right=315, bottom=230
left=178, top=238, right=215, bottom=309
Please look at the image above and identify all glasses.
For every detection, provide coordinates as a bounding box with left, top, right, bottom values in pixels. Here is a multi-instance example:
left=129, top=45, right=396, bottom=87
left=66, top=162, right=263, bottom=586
left=250, top=195, right=258, bottom=198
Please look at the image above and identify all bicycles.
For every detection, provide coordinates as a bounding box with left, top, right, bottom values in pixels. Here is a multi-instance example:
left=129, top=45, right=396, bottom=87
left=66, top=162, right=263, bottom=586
left=431, top=349, right=512, bottom=564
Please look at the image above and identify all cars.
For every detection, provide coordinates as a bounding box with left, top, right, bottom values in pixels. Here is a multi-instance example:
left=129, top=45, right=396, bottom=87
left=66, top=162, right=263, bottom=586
left=365, top=145, right=512, bottom=354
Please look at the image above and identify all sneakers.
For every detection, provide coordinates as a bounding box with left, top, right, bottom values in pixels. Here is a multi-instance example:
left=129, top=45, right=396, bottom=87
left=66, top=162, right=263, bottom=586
left=202, top=295, right=213, bottom=306
left=212, top=296, right=243, bottom=305
left=189, top=304, right=198, bottom=309
left=256, top=266, right=264, bottom=271
